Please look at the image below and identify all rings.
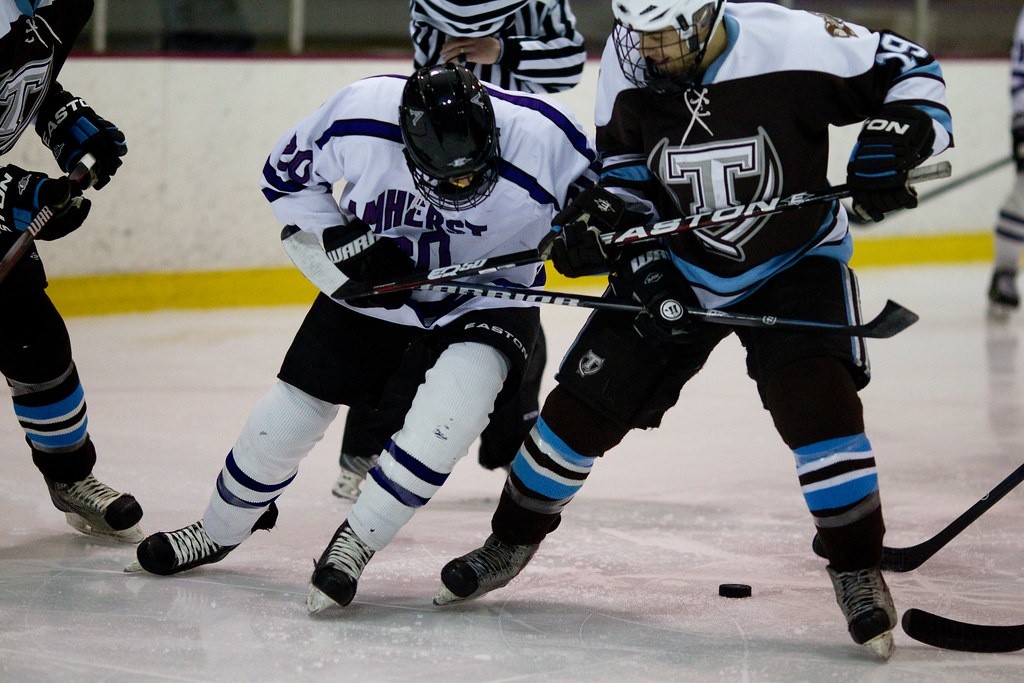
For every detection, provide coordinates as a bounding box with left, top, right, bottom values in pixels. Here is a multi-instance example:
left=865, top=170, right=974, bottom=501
left=458, top=53, right=466, bottom=63
left=460, top=48, right=464, bottom=52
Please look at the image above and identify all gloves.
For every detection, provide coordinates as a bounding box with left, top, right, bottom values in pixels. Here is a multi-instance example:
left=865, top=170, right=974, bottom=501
left=34, top=89, right=127, bottom=191
left=322, top=217, right=418, bottom=310
left=551, top=184, right=637, bottom=278
left=610, top=237, right=706, bottom=342
left=846, top=104, right=936, bottom=220
left=0, top=163, right=91, bottom=242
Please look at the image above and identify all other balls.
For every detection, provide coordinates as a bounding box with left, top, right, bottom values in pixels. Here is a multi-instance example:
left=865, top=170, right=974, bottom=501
left=718, top=584, right=751, bottom=598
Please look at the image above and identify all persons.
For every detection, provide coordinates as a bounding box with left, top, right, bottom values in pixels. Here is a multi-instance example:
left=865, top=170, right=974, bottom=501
left=430, top=0, right=953, bottom=661
left=0, top=0, right=146, bottom=544
left=331, top=0, right=586, bottom=502
left=986, top=7, right=1024, bottom=320
left=123, top=63, right=624, bottom=613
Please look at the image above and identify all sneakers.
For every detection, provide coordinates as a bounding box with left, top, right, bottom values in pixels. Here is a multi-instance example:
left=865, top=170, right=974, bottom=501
left=433, top=514, right=562, bottom=605
left=812, top=532, right=897, bottom=661
left=122, top=500, right=279, bottom=575
left=43, top=472, right=144, bottom=544
left=304, top=520, right=375, bottom=615
left=987, top=267, right=1022, bottom=319
left=332, top=453, right=382, bottom=501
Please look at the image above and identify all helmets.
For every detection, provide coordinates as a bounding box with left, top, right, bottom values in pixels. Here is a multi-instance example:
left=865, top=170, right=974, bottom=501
left=397, top=64, right=501, bottom=211
left=611, top=0, right=726, bottom=95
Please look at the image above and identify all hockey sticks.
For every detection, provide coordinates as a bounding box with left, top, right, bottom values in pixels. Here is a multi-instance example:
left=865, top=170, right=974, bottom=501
left=811, top=462, right=1023, bottom=575
left=282, top=161, right=953, bottom=341
left=916, top=153, right=1014, bottom=204
left=0, top=148, right=98, bottom=274
left=901, top=608, right=1024, bottom=655
